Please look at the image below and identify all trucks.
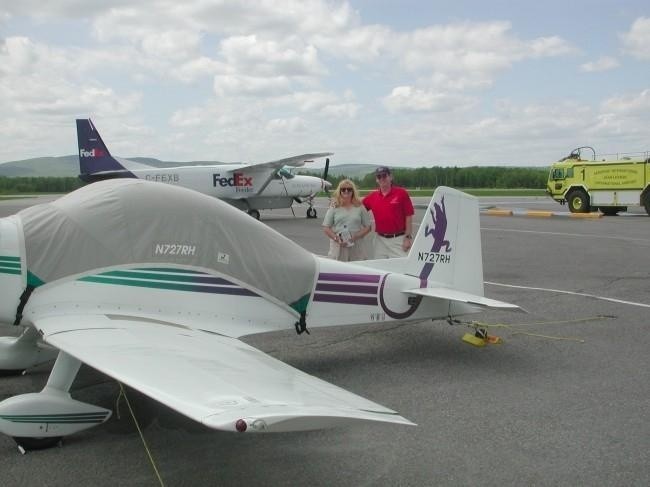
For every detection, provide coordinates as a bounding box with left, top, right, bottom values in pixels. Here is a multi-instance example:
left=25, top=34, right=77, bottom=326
left=545, top=147, right=650, bottom=215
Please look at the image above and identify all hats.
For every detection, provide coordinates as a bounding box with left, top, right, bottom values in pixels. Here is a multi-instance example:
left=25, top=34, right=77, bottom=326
left=373, top=166, right=390, bottom=177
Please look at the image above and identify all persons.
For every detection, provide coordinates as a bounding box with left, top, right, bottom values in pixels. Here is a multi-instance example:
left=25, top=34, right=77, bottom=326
left=362, top=166, right=415, bottom=259
left=322, top=179, right=373, bottom=262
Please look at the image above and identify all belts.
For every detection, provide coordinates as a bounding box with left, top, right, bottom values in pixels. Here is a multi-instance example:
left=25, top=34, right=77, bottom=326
left=379, top=232, right=405, bottom=239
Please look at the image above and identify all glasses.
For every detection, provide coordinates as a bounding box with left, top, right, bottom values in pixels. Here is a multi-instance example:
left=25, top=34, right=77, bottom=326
left=376, top=175, right=388, bottom=179
left=340, top=187, right=354, bottom=193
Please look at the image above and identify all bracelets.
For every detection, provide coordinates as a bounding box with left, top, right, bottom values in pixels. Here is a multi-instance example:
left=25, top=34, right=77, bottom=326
left=405, top=235, right=413, bottom=239
left=334, top=236, right=337, bottom=241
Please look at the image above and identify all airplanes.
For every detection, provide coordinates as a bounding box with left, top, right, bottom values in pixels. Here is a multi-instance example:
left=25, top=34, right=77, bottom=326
left=76, top=119, right=333, bottom=220
left=0, top=176, right=528, bottom=452
